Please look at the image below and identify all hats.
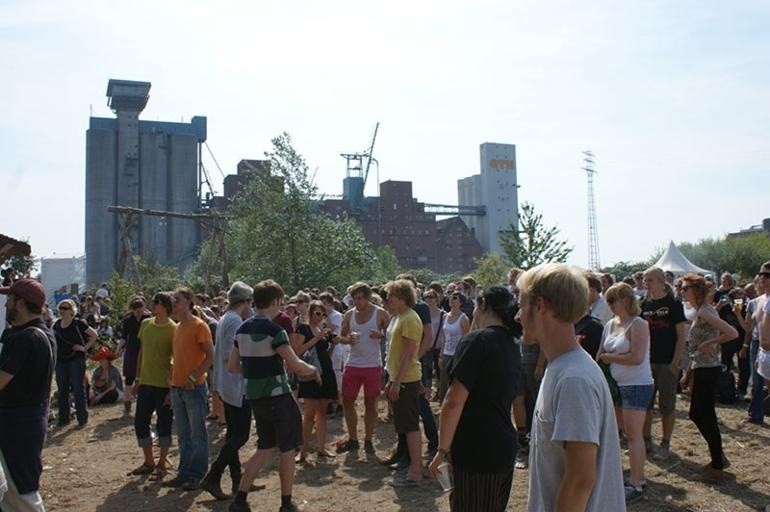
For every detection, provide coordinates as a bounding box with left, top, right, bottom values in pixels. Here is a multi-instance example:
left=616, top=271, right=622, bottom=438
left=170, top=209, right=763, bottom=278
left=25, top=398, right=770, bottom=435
left=0, top=279, right=46, bottom=307
left=88, top=346, right=120, bottom=361
left=226, top=281, right=254, bottom=303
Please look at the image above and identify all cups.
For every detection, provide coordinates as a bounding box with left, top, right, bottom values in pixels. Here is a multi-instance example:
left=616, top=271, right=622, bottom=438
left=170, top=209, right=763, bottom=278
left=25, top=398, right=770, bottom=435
left=734, top=298, right=744, bottom=311
left=351, top=331, right=362, bottom=345
left=435, top=461, right=455, bottom=492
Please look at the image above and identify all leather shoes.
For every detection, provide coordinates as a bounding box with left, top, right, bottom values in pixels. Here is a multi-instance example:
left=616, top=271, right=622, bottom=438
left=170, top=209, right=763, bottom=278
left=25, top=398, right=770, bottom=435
left=232, top=478, right=269, bottom=493
left=200, top=476, right=232, bottom=501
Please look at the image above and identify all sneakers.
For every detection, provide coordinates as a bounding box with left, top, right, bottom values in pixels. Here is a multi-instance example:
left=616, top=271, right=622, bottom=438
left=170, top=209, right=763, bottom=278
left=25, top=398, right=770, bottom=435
left=623, top=485, right=643, bottom=503
left=294, top=451, right=309, bottom=463
left=390, top=476, right=424, bottom=488
left=318, top=448, right=336, bottom=457
left=169, top=475, right=183, bottom=486
left=149, top=467, right=168, bottom=481
left=379, top=449, right=399, bottom=465
left=184, top=476, right=203, bottom=491
left=336, top=439, right=360, bottom=454
left=701, top=455, right=731, bottom=469
left=228, top=503, right=252, bottom=512
left=397, top=467, right=410, bottom=474
left=624, top=480, right=648, bottom=488
left=364, top=440, right=376, bottom=454
left=421, top=450, right=435, bottom=460
left=132, top=461, right=155, bottom=475
left=652, top=441, right=672, bottom=461
left=688, top=468, right=725, bottom=482
left=278, top=505, right=299, bottom=512
left=623, top=442, right=654, bottom=456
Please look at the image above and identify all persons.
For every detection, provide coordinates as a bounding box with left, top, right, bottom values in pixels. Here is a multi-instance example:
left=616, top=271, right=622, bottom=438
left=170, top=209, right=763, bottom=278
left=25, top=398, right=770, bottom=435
left=1, top=264, right=770, bottom=512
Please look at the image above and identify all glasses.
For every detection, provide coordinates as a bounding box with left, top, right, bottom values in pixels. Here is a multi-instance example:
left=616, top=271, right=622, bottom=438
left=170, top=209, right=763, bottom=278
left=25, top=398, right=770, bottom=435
left=449, top=295, right=459, bottom=300
left=150, top=300, right=164, bottom=305
left=758, top=272, right=770, bottom=278
left=312, top=311, right=328, bottom=318
left=423, top=295, right=435, bottom=299
left=606, top=296, right=618, bottom=304
left=295, top=300, right=308, bottom=304
left=58, top=306, right=71, bottom=310
left=680, top=285, right=695, bottom=290
